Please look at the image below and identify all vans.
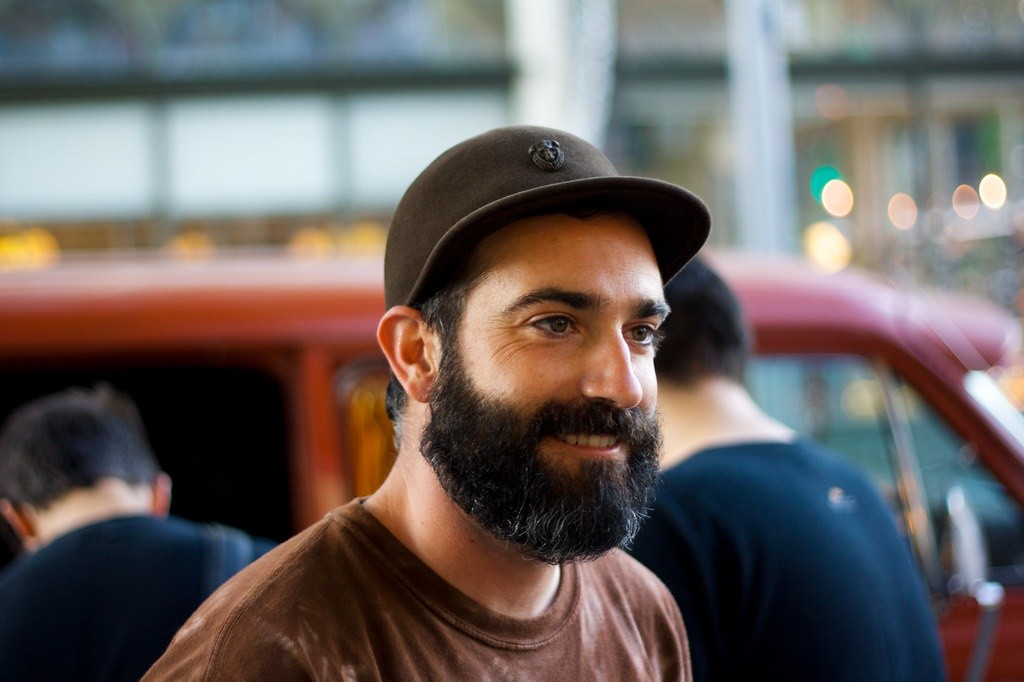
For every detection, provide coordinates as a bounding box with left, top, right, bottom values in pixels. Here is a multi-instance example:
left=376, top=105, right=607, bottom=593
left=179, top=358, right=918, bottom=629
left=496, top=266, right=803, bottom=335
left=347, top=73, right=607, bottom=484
left=0, top=253, right=1023, bottom=682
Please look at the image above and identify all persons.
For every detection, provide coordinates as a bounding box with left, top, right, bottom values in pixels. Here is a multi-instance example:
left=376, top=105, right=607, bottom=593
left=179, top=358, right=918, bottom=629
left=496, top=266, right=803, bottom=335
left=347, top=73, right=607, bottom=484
left=140, top=125, right=711, bottom=682
left=0, top=380, right=278, bottom=682
left=620, top=250, right=945, bottom=682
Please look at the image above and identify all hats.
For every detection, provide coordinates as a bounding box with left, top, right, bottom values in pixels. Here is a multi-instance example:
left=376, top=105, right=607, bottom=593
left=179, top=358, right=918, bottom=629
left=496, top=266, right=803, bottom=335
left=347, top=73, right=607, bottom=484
left=382, top=125, right=713, bottom=315
